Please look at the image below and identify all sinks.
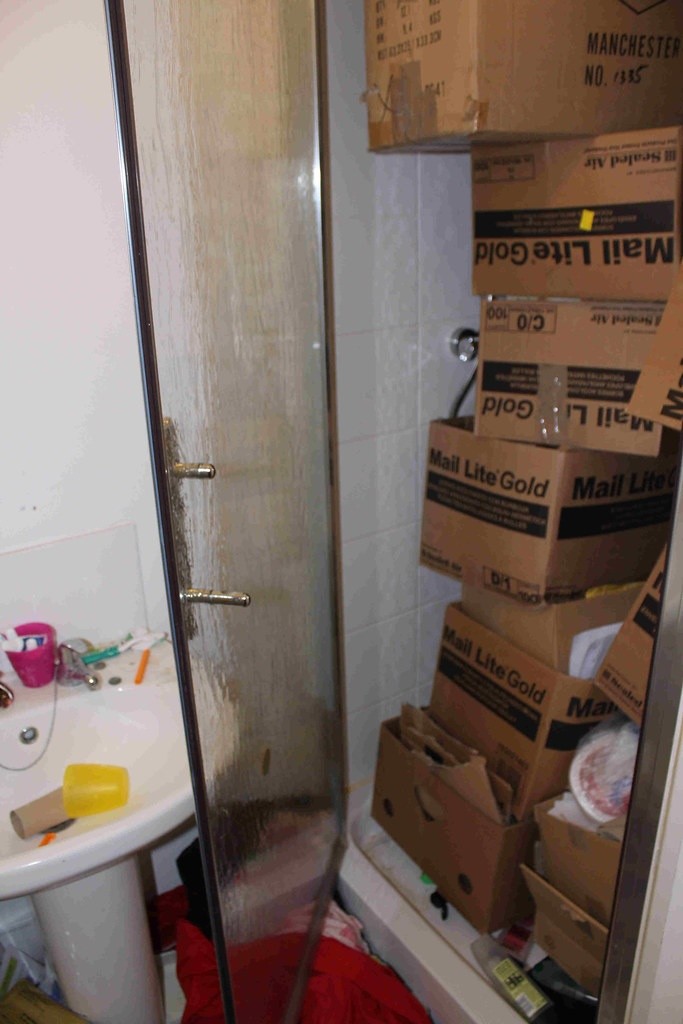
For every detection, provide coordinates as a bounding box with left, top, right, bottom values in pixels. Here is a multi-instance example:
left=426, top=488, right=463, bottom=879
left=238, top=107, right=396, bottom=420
left=1, top=637, right=241, bottom=901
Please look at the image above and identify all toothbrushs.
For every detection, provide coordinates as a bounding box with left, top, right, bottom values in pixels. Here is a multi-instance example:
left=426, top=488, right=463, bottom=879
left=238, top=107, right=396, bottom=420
left=134, top=630, right=167, bottom=683
left=82, top=632, right=135, bottom=663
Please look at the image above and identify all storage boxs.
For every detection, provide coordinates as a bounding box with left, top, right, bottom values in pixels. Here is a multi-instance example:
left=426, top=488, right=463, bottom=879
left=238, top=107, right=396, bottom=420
left=361, top=1, right=683, bottom=993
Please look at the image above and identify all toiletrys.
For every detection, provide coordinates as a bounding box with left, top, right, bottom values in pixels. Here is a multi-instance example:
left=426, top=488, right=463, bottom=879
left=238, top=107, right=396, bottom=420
left=2, top=626, right=47, bottom=654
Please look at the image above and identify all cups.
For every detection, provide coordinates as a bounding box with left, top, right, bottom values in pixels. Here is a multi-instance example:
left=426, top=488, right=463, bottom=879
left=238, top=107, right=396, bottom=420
left=3, top=622, right=57, bottom=688
left=63, top=764, right=130, bottom=817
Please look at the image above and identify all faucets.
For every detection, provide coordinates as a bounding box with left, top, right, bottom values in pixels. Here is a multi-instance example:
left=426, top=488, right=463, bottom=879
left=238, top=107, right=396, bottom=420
left=58, top=638, right=104, bottom=692
